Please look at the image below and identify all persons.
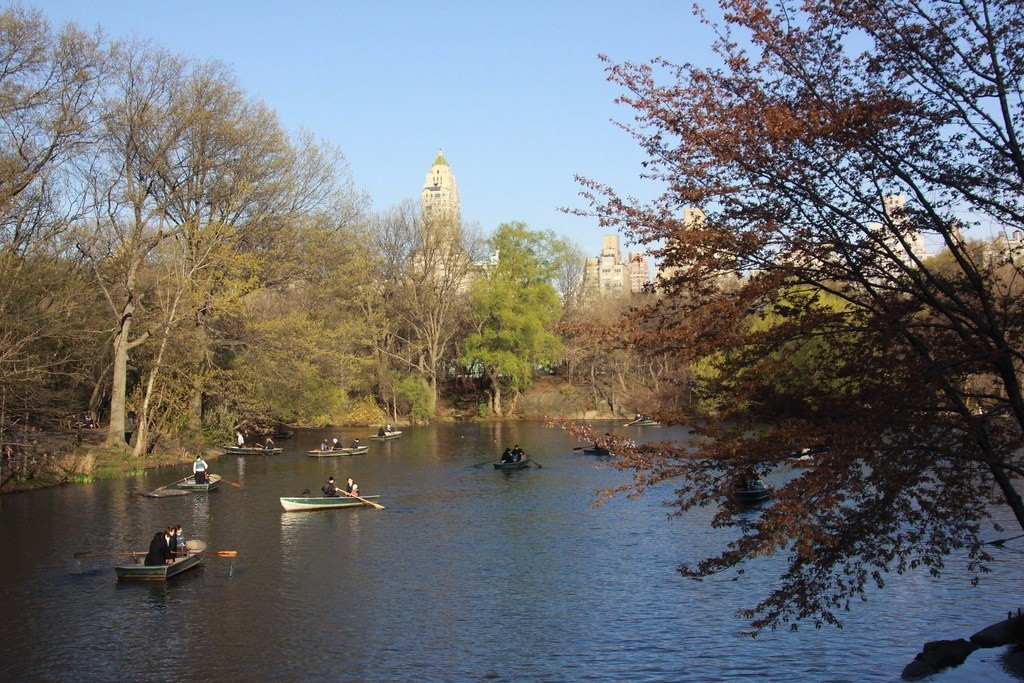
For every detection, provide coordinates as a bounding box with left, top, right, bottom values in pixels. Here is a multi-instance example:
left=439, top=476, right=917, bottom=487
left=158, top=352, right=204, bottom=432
left=320, top=438, right=329, bottom=451
left=351, top=438, right=360, bottom=448
left=264, top=438, right=274, bottom=449
left=323, top=477, right=340, bottom=497
left=332, top=438, right=343, bottom=450
left=144, top=525, right=186, bottom=566
left=386, top=424, right=392, bottom=432
left=605, top=434, right=611, bottom=446
left=193, top=456, right=208, bottom=484
left=511, top=445, right=524, bottom=462
left=752, top=475, right=765, bottom=489
left=302, top=489, right=311, bottom=497
left=378, top=426, right=386, bottom=436
left=502, top=448, right=513, bottom=463
left=236, top=431, right=244, bottom=448
left=347, top=478, right=359, bottom=497
left=635, top=414, right=640, bottom=421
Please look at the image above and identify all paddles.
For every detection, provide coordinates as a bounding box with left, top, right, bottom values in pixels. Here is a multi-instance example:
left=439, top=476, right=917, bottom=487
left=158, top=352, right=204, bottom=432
left=248, top=443, right=266, bottom=456
left=210, top=476, right=241, bottom=487
left=170, top=550, right=238, bottom=558
left=338, top=489, right=386, bottom=509
left=155, top=474, right=195, bottom=492
left=74, top=550, right=149, bottom=559
left=331, top=448, right=353, bottom=450
left=481, top=457, right=502, bottom=467
left=623, top=419, right=641, bottom=427
left=573, top=444, right=594, bottom=451
left=523, top=454, right=541, bottom=467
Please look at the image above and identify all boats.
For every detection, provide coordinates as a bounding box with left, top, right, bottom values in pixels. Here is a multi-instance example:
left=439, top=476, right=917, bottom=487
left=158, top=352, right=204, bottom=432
left=177, top=473, right=223, bottom=493
left=732, top=482, right=776, bottom=502
left=279, top=494, right=381, bottom=512
left=493, top=454, right=532, bottom=470
left=583, top=445, right=611, bottom=456
left=114, top=539, right=208, bottom=581
left=306, top=445, right=370, bottom=458
left=631, top=419, right=661, bottom=426
left=369, top=430, right=403, bottom=441
left=222, top=445, right=283, bottom=456
